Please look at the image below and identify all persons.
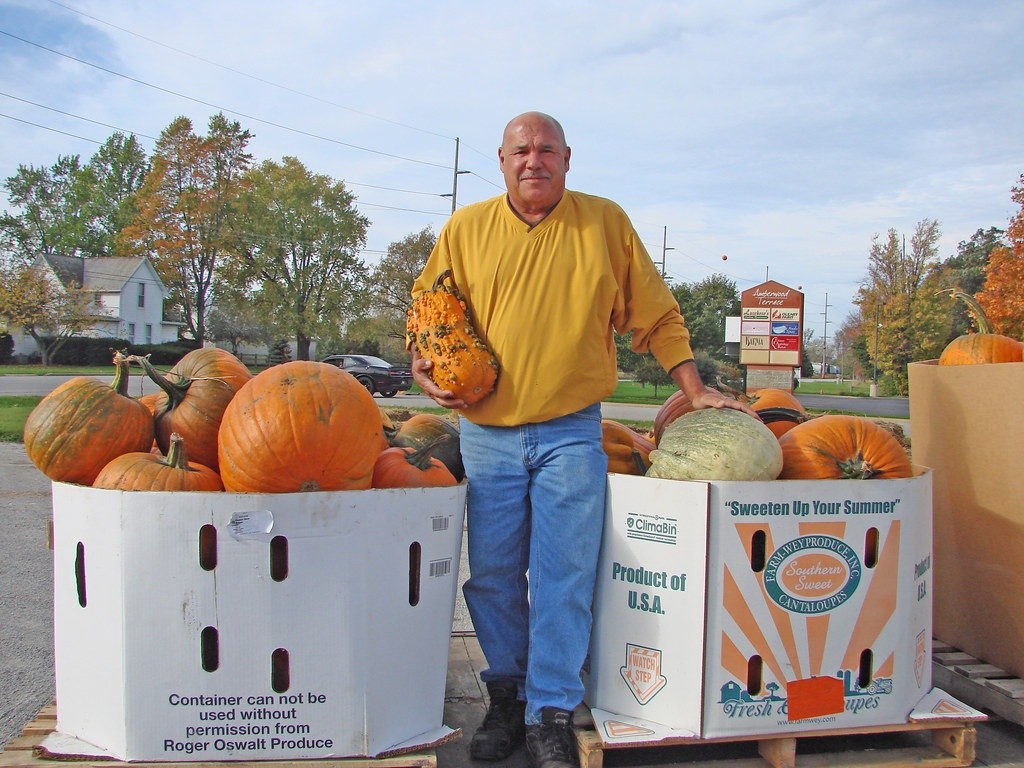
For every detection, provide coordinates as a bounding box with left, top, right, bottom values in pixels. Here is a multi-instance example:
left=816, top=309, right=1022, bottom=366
left=398, top=111, right=765, bottom=768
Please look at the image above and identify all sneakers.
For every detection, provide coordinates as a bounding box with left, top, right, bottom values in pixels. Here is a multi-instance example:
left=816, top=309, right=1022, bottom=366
left=471, top=679, right=527, bottom=761
left=525, top=706, right=580, bottom=768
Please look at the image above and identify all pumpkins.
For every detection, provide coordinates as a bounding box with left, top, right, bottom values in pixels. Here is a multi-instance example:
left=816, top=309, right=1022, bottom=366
left=599, top=383, right=912, bottom=567
left=407, top=270, right=499, bottom=405
left=938, top=291, right=1024, bottom=365
left=24, top=349, right=467, bottom=569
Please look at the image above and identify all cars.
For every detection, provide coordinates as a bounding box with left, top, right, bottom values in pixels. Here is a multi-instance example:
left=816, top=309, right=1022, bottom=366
left=321, top=355, right=414, bottom=398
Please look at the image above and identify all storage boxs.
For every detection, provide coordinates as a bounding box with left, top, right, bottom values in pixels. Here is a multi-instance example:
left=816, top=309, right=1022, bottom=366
left=50, top=476, right=468, bottom=764
left=580, top=467, right=935, bottom=737
left=906, top=359, right=1024, bottom=678
